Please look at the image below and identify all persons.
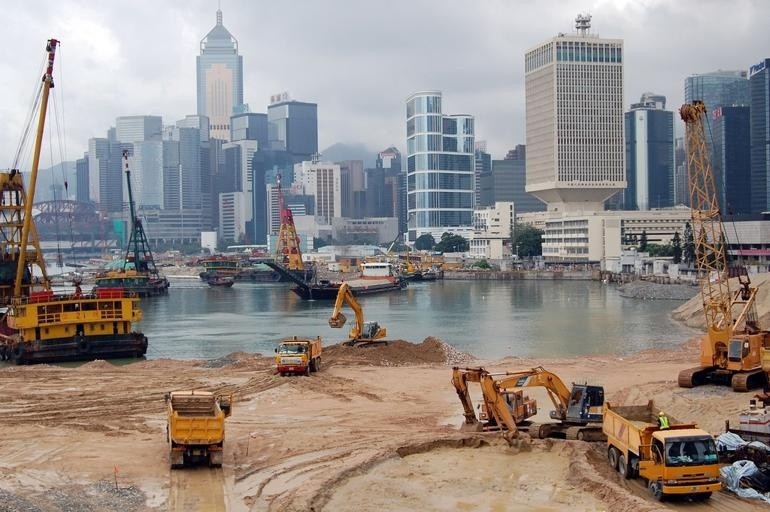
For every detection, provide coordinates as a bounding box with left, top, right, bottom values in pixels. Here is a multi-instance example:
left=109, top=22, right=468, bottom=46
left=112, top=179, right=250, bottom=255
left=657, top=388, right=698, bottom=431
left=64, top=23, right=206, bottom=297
left=656, top=411, right=670, bottom=430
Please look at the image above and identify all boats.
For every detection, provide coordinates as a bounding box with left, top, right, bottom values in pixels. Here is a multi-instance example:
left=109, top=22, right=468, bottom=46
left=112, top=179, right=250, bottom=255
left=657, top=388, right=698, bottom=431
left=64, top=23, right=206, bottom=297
left=199, top=173, right=442, bottom=300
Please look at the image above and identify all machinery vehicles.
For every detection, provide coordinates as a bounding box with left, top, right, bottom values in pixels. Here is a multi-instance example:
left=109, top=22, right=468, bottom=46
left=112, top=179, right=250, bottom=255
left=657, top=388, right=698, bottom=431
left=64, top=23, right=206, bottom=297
left=329, top=283, right=388, bottom=349
left=451, top=366, right=607, bottom=455
left=679, top=99, right=770, bottom=392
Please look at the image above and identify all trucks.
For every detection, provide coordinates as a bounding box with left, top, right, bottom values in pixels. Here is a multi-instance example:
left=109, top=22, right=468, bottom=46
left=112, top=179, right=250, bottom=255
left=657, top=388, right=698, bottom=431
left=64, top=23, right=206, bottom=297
left=601, top=400, right=722, bottom=502
left=164, top=389, right=233, bottom=470
left=274, top=335, right=322, bottom=376
